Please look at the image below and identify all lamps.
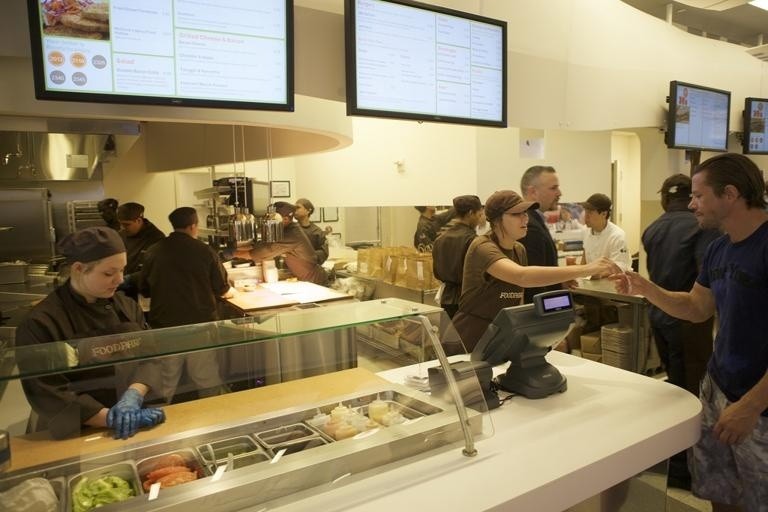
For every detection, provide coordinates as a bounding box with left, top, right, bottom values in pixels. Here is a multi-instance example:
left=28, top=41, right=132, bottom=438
left=227, top=124, right=285, bottom=244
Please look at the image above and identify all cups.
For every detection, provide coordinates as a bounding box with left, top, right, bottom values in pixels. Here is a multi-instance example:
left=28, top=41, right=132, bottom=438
left=566, top=256, right=576, bottom=266
left=261, top=259, right=279, bottom=284
left=0, top=428, right=10, bottom=471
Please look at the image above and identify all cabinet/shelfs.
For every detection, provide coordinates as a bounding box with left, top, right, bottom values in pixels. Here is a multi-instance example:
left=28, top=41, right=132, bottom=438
left=1, top=295, right=494, bottom=511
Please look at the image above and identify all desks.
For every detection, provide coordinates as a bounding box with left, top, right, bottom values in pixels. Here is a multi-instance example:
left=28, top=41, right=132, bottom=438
left=564, top=276, right=669, bottom=381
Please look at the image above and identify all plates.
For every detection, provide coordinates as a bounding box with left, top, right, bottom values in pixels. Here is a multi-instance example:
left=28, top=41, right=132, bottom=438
left=233, top=263, right=251, bottom=267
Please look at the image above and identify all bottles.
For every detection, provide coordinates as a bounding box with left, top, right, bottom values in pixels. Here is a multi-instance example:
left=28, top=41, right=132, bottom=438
left=347, top=282, right=365, bottom=299
left=311, top=392, right=407, bottom=442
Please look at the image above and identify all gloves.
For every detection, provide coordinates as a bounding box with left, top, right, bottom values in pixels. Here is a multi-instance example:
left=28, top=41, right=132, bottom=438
left=107, top=389, right=165, bottom=440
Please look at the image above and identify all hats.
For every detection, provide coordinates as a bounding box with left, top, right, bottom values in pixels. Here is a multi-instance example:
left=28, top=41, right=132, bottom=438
left=453, top=174, right=692, bottom=220
left=98, top=199, right=314, bottom=227
left=58, top=227, right=127, bottom=262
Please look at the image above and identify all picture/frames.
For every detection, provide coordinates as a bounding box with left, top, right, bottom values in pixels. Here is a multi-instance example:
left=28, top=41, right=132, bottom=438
left=308, top=205, right=343, bottom=239
left=267, top=178, right=292, bottom=199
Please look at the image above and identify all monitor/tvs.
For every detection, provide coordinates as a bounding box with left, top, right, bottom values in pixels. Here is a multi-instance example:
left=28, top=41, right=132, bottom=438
left=742, top=97, right=768, bottom=155
left=467, top=303, right=577, bottom=400
left=344, top=0, right=508, bottom=128
left=246, top=179, right=272, bottom=217
left=27, top=0, right=295, bottom=112
left=667, top=81, right=731, bottom=153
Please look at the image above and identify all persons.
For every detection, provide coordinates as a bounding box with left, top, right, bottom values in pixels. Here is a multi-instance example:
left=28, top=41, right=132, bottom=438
left=96, top=198, right=166, bottom=302
left=219, top=199, right=330, bottom=288
left=136, top=206, right=230, bottom=401
left=14, top=227, right=166, bottom=440
left=415, top=166, right=635, bottom=358
left=606, top=152, right=767, bottom=509
